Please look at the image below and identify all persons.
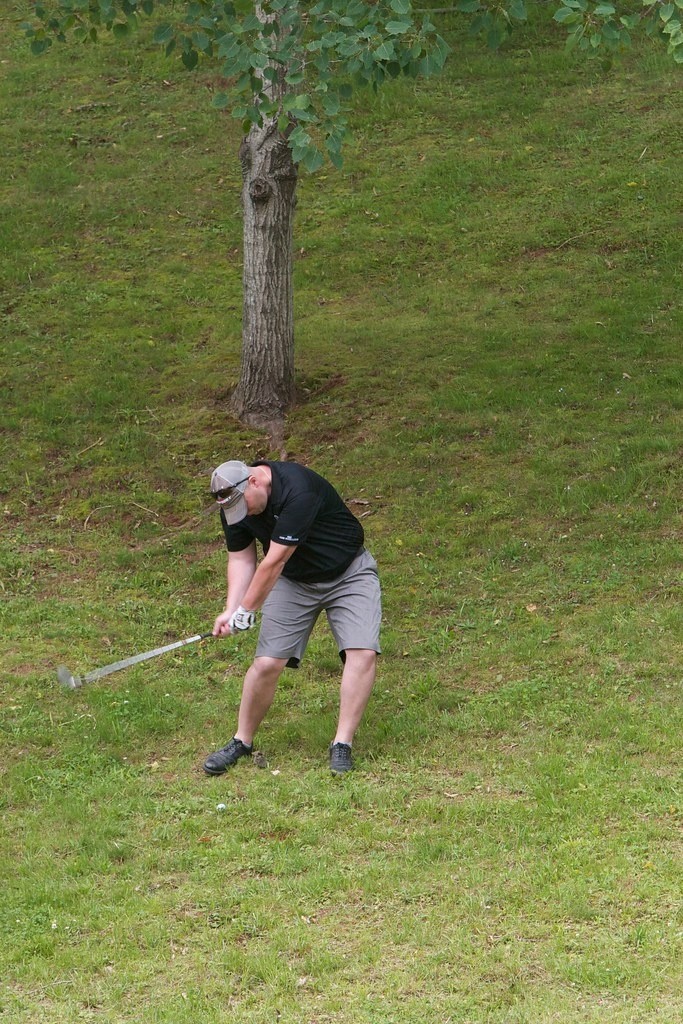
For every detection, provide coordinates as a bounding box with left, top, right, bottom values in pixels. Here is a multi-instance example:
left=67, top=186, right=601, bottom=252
left=203, top=460, right=384, bottom=775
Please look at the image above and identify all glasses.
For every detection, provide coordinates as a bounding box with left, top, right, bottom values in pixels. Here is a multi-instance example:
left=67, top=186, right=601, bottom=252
left=210, top=475, right=252, bottom=500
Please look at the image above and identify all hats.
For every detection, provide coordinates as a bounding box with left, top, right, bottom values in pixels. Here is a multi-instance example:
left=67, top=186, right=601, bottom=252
left=210, top=459, right=251, bottom=526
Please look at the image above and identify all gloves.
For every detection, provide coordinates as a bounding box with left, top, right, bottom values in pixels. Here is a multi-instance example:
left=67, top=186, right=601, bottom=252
left=228, top=605, right=257, bottom=635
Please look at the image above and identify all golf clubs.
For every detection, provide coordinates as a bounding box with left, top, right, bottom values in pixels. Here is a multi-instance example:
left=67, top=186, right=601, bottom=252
left=56, top=631, right=210, bottom=691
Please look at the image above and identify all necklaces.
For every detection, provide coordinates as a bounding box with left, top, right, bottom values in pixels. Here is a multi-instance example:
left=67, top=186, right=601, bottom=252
left=258, top=467, right=271, bottom=483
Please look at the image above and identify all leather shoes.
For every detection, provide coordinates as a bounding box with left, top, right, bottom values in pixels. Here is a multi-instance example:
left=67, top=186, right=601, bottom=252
left=329, top=740, right=353, bottom=776
left=201, top=736, right=253, bottom=775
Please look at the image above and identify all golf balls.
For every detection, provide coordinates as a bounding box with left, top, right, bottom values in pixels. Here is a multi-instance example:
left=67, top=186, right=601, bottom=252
left=216, top=803, right=226, bottom=809
